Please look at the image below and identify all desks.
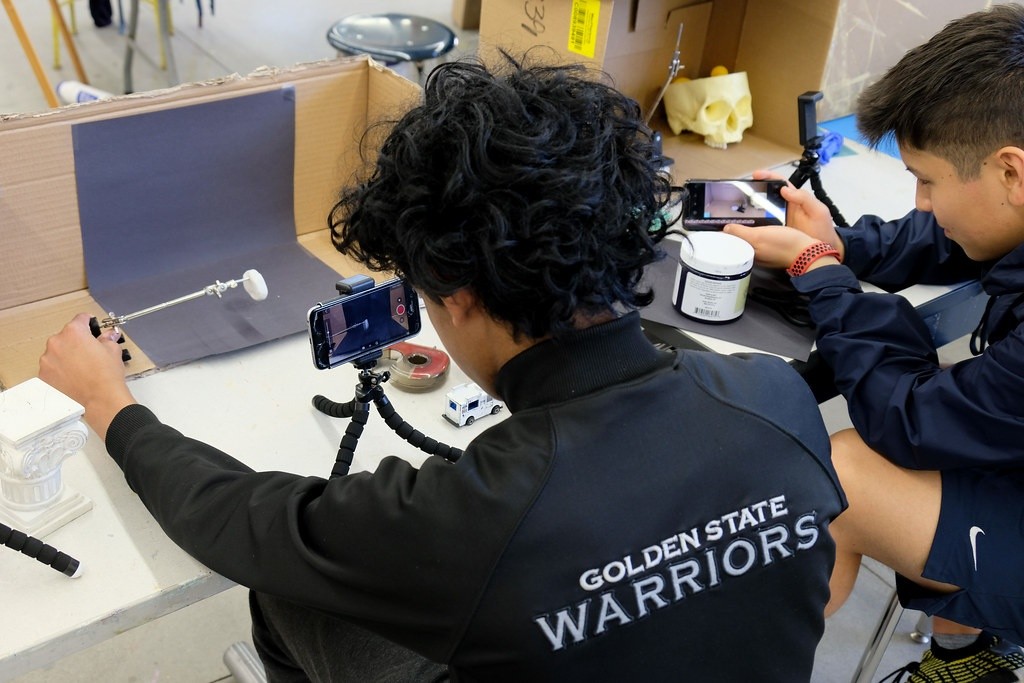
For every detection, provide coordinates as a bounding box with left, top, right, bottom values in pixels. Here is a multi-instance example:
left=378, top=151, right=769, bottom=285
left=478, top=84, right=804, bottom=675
left=0, top=125, right=991, bottom=682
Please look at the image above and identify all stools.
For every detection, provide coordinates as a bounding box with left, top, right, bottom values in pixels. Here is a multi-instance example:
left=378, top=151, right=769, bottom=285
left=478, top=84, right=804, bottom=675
left=325, top=9, right=459, bottom=86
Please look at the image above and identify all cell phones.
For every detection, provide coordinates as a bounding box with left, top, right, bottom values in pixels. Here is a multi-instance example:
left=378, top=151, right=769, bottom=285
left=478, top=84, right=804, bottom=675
left=306, top=274, right=421, bottom=369
left=681, top=179, right=788, bottom=231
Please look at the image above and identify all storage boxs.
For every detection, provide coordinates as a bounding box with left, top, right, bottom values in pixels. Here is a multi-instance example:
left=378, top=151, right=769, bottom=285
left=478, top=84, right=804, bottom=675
left=477, top=0, right=842, bottom=209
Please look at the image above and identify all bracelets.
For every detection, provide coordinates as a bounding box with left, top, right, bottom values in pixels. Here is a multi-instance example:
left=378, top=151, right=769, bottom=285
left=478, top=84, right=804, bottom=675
left=787, top=241, right=841, bottom=277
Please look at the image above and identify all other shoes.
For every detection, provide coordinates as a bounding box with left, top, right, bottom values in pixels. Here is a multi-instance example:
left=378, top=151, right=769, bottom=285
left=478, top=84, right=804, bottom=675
left=875, top=631, right=1024, bottom=683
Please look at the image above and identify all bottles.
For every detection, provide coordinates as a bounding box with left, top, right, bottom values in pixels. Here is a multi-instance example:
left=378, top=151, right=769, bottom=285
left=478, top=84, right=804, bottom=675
left=56, top=80, right=113, bottom=104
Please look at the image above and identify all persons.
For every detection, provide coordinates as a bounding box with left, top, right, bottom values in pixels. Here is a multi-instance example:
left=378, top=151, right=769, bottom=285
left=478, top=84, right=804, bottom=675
left=722, top=4, right=1024, bottom=683
left=36, top=48, right=849, bottom=683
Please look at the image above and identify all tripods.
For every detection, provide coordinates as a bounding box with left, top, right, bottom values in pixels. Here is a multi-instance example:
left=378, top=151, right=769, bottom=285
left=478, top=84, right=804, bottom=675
left=312, top=369, right=466, bottom=480
left=788, top=149, right=851, bottom=227
